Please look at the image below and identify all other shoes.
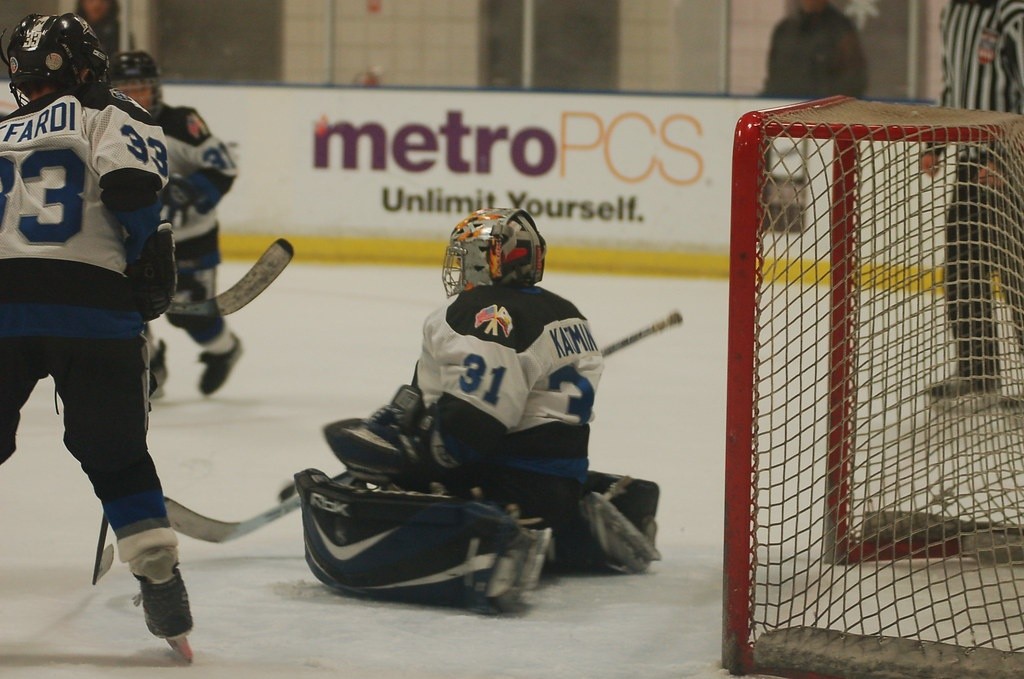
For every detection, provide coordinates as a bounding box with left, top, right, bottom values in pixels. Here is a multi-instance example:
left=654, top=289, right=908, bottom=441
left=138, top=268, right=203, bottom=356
left=929, top=377, right=1002, bottom=414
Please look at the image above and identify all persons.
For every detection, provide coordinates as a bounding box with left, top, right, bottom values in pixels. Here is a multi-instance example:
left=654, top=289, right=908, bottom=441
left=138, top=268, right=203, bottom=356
left=110, top=51, right=244, bottom=394
left=764, top=0, right=868, bottom=99
left=78, top=0, right=134, bottom=57
left=0, top=13, right=193, bottom=663
left=920, top=0, right=1024, bottom=409
left=417, top=209, right=604, bottom=563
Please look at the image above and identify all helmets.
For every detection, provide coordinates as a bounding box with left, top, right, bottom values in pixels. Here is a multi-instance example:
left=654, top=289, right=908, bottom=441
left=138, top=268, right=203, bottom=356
left=105, top=51, right=160, bottom=89
left=449, top=207, right=548, bottom=289
left=7, top=13, right=108, bottom=88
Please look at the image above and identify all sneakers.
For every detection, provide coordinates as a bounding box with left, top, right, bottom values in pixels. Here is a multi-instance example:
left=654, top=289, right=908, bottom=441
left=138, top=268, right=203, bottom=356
left=198, top=331, right=243, bottom=395
left=134, top=561, right=195, bottom=663
left=149, top=341, right=168, bottom=401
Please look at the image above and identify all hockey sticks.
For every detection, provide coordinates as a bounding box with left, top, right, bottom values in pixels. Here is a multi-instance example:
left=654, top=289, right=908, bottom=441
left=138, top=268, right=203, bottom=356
left=91, top=515, right=118, bottom=585
left=164, top=312, right=685, bottom=545
left=170, top=238, right=294, bottom=319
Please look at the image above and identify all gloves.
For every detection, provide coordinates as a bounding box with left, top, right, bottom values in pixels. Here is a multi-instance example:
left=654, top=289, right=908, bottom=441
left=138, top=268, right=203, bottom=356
left=134, top=218, right=177, bottom=321
left=162, top=178, right=195, bottom=209
